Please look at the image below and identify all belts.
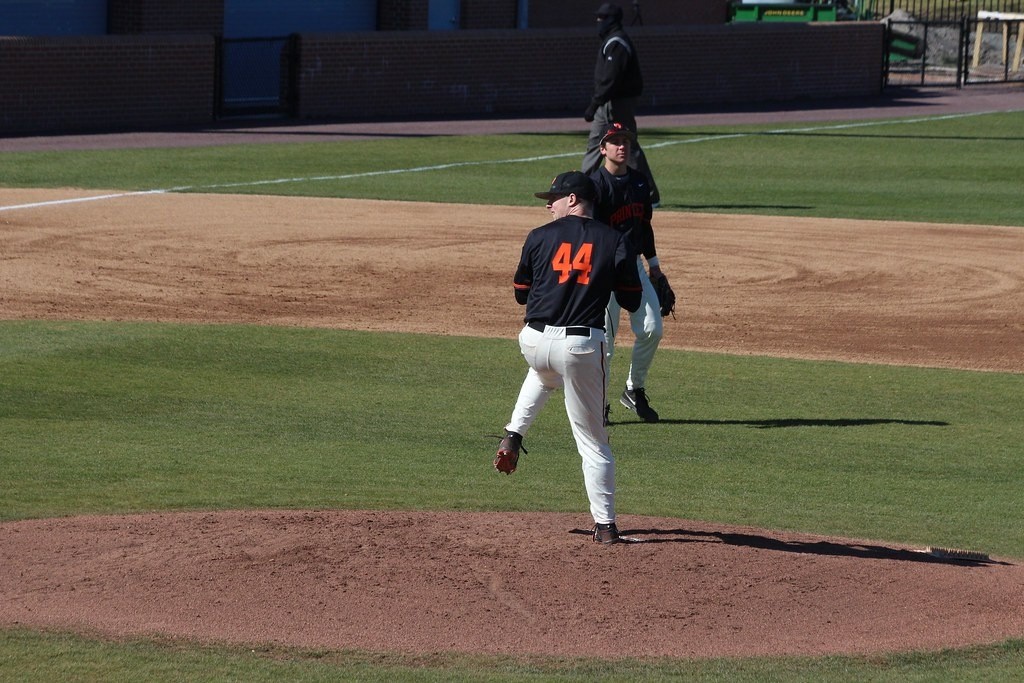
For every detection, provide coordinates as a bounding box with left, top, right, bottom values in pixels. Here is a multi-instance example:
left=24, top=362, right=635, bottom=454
left=528, top=321, right=590, bottom=336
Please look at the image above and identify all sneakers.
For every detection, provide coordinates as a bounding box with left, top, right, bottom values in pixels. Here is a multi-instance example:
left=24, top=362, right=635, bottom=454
left=604, top=404, right=610, bottom=426
left=619, top=385, right=659, bottom=423
left=486, top=428, right=527, bottom=475
left=591, top=523, right=620, bottom=544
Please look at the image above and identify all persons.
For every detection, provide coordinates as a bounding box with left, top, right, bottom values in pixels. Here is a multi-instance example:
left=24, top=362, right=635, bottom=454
left=494, top=171, right=642, bottom=542
left=581, top=2, right=661, bottom=207
left=585, top=122, right=662, bottom=423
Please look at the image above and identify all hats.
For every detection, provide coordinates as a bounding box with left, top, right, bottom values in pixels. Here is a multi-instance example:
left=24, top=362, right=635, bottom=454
left=534, top=170, right=597, bottom=202
left=599, top=120, right=634, bottom=146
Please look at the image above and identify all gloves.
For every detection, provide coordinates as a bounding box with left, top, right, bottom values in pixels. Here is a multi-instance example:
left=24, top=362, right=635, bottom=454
left=585, top=105, right=598, bottom=122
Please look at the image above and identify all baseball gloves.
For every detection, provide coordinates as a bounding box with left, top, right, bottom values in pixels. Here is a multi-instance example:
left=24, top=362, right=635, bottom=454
left=650, top=273, right=675, bottom=318
